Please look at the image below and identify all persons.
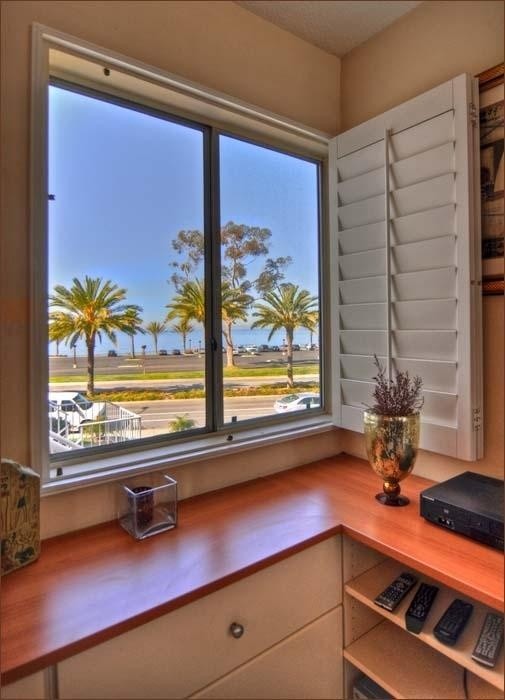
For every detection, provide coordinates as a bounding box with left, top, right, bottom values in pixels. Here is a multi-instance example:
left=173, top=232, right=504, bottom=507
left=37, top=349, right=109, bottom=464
left=11, top=474, right=31, bottom=530
left=1, top=483, right=9, bottom=535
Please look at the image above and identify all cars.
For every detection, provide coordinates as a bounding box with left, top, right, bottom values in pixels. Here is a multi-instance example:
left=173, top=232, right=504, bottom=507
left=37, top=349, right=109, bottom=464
left=108, top=350, right=117, bottom=357
left=159, top=349, right=180, bottom=355
left=274, top=392, right=320, bottom=412
left=232, top=344, right=316, bottom=355
left=49, top=391, right=106, bottom=433
left=222, top=347, right=226, bottom=353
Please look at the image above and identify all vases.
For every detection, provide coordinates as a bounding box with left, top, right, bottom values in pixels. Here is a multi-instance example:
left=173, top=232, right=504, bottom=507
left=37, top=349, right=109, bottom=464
left=364, top=411, right=419, bottom=505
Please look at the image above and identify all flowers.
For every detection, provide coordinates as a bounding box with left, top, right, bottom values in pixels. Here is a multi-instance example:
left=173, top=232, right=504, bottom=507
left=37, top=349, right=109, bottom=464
left=364, top=354, right=426, bottom=481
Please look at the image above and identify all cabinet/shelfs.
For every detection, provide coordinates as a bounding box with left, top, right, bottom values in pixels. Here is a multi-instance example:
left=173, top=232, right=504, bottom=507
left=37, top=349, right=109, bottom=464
left=341, top=557, right=505, bottom=699
left=53, top=531, right=344, bottom=700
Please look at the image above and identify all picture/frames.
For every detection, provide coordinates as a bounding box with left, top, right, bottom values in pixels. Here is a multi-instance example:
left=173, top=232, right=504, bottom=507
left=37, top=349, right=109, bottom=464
left=476, top=59, right=505, bottom=294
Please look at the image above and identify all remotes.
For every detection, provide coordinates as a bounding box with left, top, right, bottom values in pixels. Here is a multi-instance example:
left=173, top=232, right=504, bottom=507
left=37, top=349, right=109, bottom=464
left=405, top=582, right=440, bottom=621
left=432, top=598, right=474, bottom=646
left=472, top=612, right=505, bottom=668
left=373, top=570, right=419, bottom=611
